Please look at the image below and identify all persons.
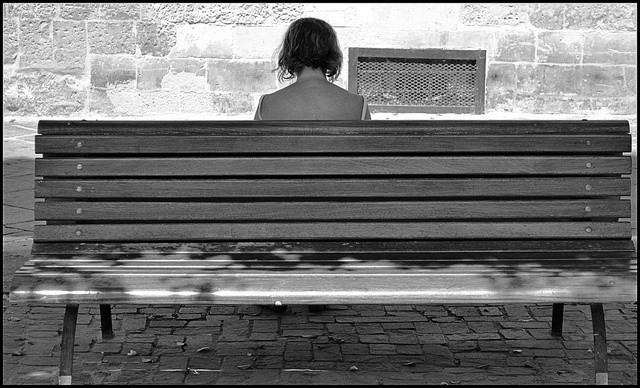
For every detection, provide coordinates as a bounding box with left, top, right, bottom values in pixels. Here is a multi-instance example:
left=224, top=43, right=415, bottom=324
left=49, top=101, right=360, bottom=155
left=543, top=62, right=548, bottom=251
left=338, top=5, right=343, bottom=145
left=252, top=18, right=372, bottom=121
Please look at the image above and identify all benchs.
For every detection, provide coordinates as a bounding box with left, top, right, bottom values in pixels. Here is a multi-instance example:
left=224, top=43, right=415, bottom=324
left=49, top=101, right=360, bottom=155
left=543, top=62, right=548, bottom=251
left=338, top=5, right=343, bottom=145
left=8, top=121, right=637, bottom=385
